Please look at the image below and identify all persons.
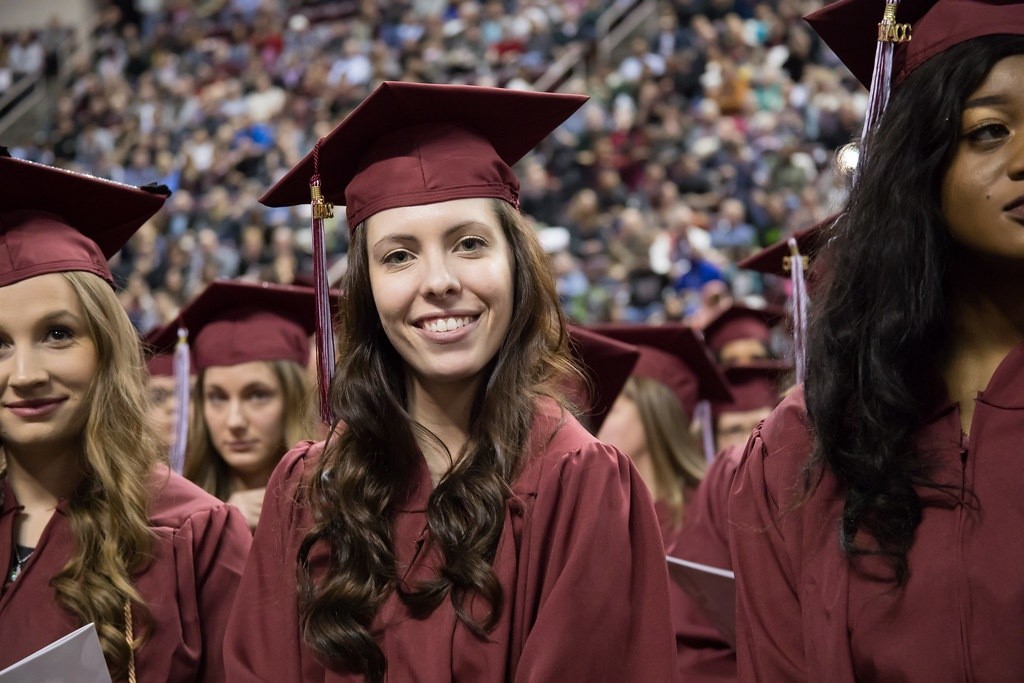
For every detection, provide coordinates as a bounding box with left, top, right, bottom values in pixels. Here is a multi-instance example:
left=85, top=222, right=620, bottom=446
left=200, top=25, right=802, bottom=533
left=726, top=0, right=1024, bottom=683
left=145, top=280, right=345, bottom=535
left=708, top=362, right=791, bottom=456
left=0, top=145, right=254, bottom=682
left=585, top=326, right=733, bottom=548
left=221, top=80, right=680, bottom=683
left=667, top=209, right=837, bottom=683
left=0, top=0, right=869, bottom=342
left=702, top=305, right=784, bottom=362
left=561, top=321, right=641, bottom=436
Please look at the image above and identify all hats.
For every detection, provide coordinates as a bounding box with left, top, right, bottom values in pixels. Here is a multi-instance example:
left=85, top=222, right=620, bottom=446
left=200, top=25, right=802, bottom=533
left=531, top=313, right=642, bottom=437
left=1, top=156, right=170, bottom=290
left=801, top=0, right=1024, bottom=190
left=585, top=326, right=737, bottom=427
left=258, top=81, right=591, bottom=425
left=737, top=212, right=844, bottom=383
left=141, top=280, right=348, bottom=473
left=703, top=303, right=781, bottom=357
left=712, top=363, right=795, bottom=413
